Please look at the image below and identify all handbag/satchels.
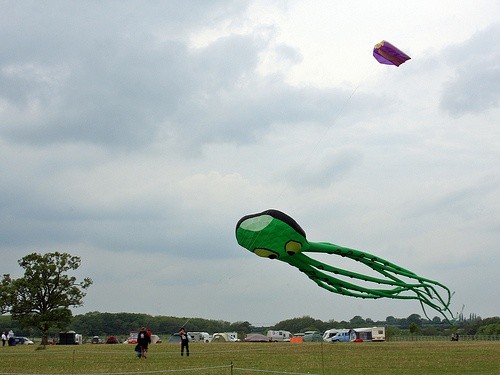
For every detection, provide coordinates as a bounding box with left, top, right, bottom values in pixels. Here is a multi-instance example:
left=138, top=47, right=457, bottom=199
left=135, top=344, right=141, bottom=353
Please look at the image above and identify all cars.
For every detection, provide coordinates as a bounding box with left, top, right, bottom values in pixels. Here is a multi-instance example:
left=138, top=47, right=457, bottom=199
left=226, top=336, right=241, bottom=342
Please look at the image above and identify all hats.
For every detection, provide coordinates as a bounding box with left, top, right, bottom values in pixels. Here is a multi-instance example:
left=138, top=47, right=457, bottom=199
left=140, top=327, right=146, bottom=331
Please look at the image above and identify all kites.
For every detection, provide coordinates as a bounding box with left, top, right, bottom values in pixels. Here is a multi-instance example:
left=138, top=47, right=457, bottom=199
left=234, top=208, right=455, bottom=324
left=373, top=39, right=410, bottom=67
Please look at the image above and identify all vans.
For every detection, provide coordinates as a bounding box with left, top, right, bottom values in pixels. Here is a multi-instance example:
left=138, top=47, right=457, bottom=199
left=267, top=330, right=294, bottom=342
left=10, top=337, right=34, bottom=345
left=332, top=333, right=356, bottom=342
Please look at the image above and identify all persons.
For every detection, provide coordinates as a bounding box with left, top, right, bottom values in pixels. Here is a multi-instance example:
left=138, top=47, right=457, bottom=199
left=135, top=344, right=142, bottom=358
left=174, top=328, right=190, bottom=358
left=2, top=329, right=14, bottom=346
left=137, top=327, right=151, bottom=359
